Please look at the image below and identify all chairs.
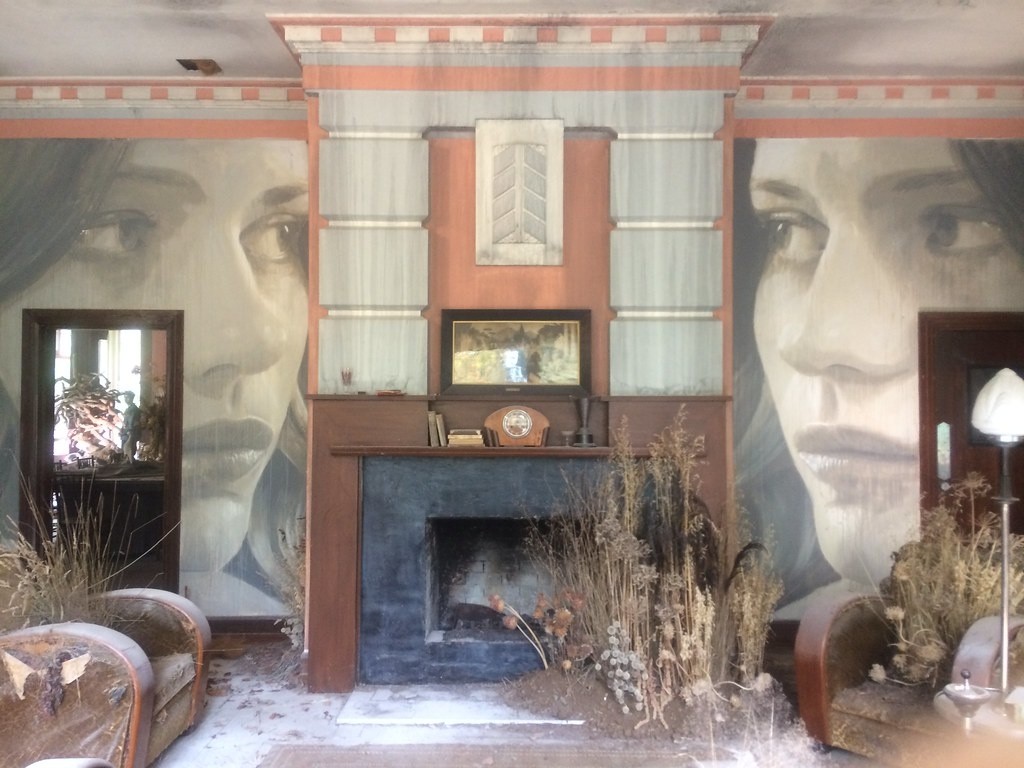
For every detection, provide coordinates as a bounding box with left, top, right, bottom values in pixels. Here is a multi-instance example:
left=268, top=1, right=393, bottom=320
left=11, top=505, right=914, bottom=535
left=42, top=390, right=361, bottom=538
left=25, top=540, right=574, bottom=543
left=51, top=455, right=104, bottom=547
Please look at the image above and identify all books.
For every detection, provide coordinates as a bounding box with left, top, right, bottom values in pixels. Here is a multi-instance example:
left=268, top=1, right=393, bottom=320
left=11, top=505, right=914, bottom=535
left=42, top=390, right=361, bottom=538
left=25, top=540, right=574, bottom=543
left=428, top=411, right=485, bottom=447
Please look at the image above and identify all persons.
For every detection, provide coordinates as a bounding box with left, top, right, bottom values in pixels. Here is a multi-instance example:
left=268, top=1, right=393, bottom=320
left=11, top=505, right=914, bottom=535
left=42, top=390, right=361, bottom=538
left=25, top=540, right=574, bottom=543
left=117, top=391, right=143, bottom=463
left=0, top=139, right=1024, bottom=616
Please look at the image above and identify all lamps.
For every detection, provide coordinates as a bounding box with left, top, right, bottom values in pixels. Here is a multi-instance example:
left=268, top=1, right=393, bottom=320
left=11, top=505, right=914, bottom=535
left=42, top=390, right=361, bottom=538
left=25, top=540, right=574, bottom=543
left=932, top=369, right=1024, bottom=744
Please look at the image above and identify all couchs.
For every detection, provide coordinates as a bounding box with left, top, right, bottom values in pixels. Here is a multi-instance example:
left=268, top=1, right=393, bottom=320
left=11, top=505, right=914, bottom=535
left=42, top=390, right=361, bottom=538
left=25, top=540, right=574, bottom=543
left=0, top=550, right=212, bottom=768
left=796, top=542, right=1024, bottom=768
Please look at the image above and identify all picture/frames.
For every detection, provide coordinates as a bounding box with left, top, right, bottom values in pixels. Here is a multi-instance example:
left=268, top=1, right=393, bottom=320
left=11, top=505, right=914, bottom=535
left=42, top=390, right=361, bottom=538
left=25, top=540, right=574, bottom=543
left=440, top=308, right=592, bottom=397
